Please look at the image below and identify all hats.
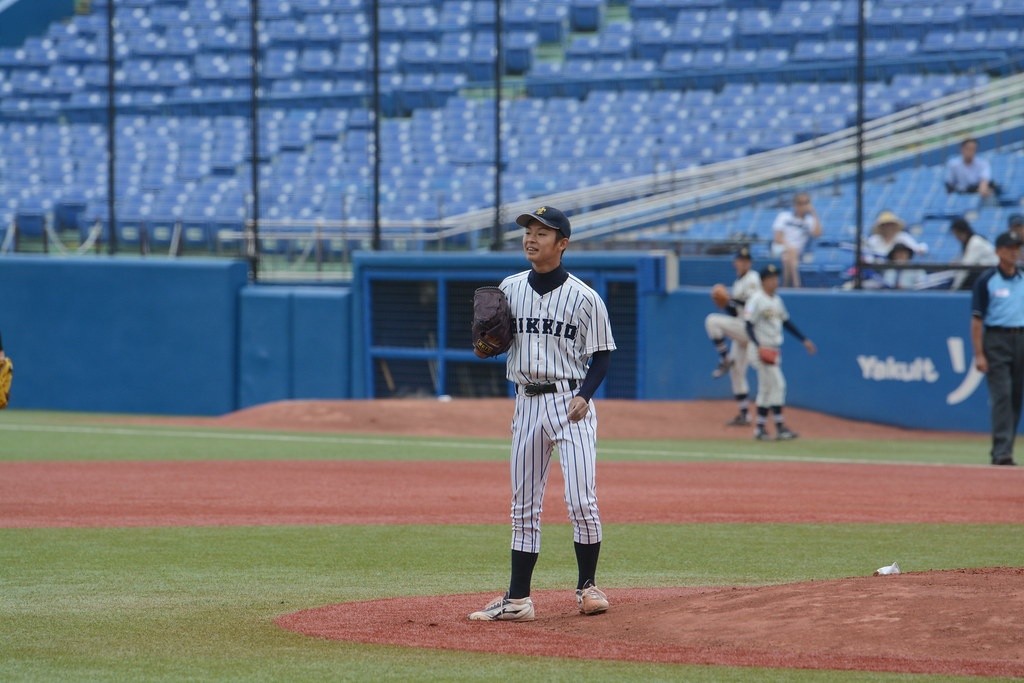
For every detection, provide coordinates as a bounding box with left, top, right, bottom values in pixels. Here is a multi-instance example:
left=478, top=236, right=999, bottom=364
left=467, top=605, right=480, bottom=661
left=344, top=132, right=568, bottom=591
left=996, top=231, right=1024, bottom=245
left=1009, top=215, right=1024, bottom=226
left=761, top=265, right=784, bottom=279
left=888, top=244, right=914, bottom=260
left=736, top=246, right=754, bottom=259
left=516, top=206, right=571, bottom=239
left=874, top=212, right=905, bottom=233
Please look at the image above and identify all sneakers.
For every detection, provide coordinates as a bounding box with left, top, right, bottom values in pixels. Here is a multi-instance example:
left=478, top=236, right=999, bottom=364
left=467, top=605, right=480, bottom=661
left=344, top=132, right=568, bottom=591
left=466, top=590, right=535, bottom=621
left=575, top=579, right=608, bottom=614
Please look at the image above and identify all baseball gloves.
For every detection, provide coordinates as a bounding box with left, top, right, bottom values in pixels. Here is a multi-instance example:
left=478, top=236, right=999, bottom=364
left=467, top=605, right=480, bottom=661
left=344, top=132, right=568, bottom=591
left=759, top=345, right=780, bottom=365
left=472, top=287, right=514, bottom=358
left=0, top=357, right=13, bottom=409
left=712, top=283, right=731, bottom=308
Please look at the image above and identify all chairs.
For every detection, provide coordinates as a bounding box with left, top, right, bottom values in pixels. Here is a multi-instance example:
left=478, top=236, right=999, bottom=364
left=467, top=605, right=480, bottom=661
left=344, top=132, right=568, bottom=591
left=0, top=0, right=1023, bottom=292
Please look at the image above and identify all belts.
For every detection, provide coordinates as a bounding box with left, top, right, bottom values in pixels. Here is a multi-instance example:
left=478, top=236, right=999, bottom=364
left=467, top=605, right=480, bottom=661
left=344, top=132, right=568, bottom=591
left=986, top=326, right=1024, bottom=333
left=516, top=380, right=577, bottom=396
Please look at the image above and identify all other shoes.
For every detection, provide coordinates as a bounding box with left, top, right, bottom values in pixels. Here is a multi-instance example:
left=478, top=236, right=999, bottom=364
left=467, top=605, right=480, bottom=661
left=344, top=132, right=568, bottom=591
left=728, top=416, right=751, bottom=425
left=714, top=360, right=733, bottom=377
left=756, top=428, right=768, bottom=440
left=777, top=431, right=796, bottom=439
left=992, top=458, right=1016, bottom=466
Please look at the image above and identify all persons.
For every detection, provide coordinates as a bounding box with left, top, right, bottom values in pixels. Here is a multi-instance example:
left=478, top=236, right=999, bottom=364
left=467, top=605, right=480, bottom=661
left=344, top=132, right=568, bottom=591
left=742, top=264, right=818, bottom=440
left=0, top=333, right=13, bottom=410
left=842, top=242, right=929, bottom=290
left=771, top=191, right=823, bottom=288
left=1009, top=211, right=1024, bottom=235
left=947, top=220, right=1002, bottom=291
left=468, top=205, right=617, bottom=623
left=860, top=211, right=929, bottom=258
left=944, top=138, right=1002, bottom=197
left=704, top=252, right=762, bottom=426
left=971, top=230, right=1024, bottom=466
